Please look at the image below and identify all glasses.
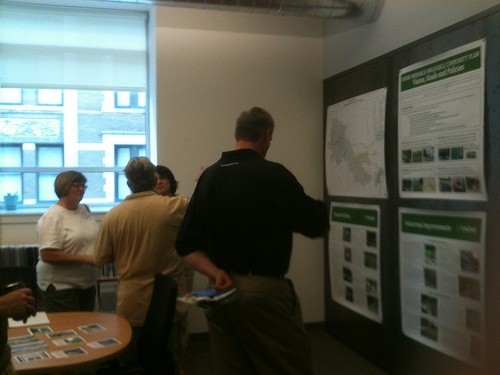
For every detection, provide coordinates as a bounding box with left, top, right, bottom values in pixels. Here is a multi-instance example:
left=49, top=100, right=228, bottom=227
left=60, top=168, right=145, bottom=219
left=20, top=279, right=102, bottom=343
left=72, top=182, right=88, bottom=190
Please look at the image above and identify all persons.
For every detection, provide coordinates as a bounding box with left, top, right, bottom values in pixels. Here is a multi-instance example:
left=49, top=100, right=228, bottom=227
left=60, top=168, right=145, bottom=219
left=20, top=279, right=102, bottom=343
left=93, top=157, right=195, bottom=375
left=0, top=287, right=34, bottom=375
left=36, top=170, right=100, bottom=312
left=175, top=106, right=330, bottom=375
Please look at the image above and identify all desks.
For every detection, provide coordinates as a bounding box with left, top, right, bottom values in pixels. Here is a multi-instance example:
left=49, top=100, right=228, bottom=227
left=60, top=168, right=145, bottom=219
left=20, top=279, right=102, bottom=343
left=8, top=312, right=132, bottom=375
